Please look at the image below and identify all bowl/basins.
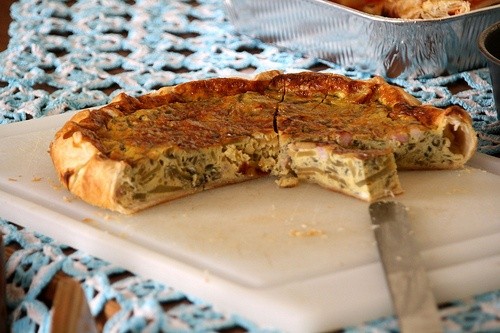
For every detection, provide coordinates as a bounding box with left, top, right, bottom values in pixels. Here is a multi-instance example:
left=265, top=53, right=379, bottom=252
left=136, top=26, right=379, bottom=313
left=478, top=22, right=500, bottom=121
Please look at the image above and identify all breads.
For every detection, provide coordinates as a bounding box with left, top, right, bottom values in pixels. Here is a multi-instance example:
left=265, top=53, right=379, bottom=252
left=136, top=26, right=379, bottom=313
left=331, top=0, right=500, bottom=20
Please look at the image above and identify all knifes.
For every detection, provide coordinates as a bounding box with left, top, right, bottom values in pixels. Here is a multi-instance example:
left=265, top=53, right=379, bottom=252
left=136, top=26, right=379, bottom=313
left=369, top=201, right=443, bottom=333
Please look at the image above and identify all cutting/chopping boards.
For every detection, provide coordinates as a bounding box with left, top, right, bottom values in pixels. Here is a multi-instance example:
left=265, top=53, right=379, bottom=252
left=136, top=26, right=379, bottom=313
left=0, top=104, right=500, bottom=333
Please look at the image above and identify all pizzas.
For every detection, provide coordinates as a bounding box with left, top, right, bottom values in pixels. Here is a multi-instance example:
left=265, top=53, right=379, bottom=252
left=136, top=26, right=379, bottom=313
left=48, top=72, right=477, bottom=214
